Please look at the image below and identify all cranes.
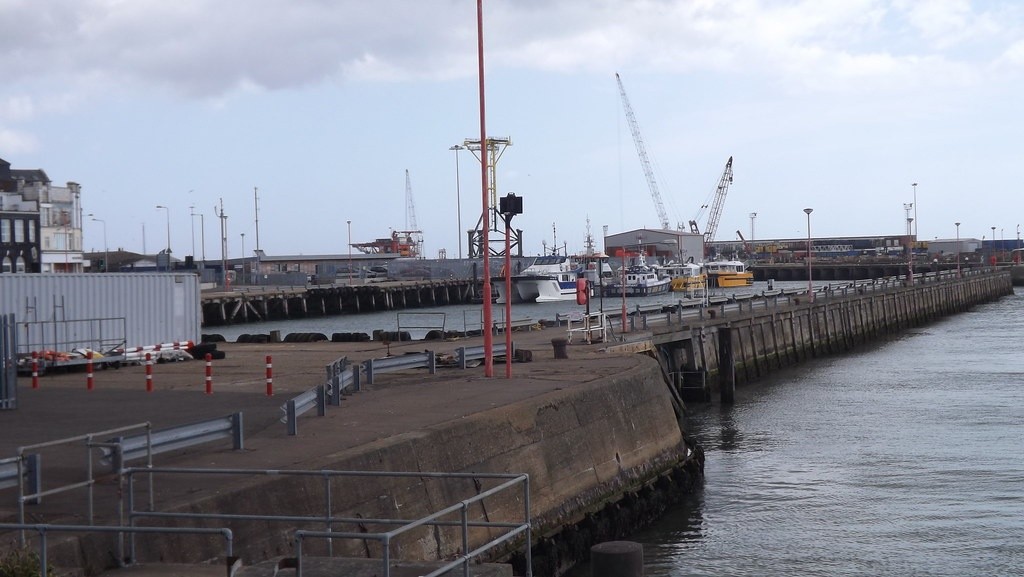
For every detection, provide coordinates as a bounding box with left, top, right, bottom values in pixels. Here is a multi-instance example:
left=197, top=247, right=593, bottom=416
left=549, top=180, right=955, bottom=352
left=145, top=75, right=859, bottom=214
left=613, top=72, right=737, bottom=263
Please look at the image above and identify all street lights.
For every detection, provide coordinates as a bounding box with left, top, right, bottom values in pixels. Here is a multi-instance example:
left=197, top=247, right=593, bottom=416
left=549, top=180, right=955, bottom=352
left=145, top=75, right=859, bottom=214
left=953, top=221, right=963, bottom=280
left=990, top=226, right=998, bottom=273
left=906, top=215, right=913, bottom=284
left=805, top=207, right=815, bottom=301
left=1017, top=231, right=1021, bottom=265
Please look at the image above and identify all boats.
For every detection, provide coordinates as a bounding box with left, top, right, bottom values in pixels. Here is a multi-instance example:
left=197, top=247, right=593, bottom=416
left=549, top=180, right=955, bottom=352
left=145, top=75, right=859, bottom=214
left=491, top=236, right=754, bottom=304
